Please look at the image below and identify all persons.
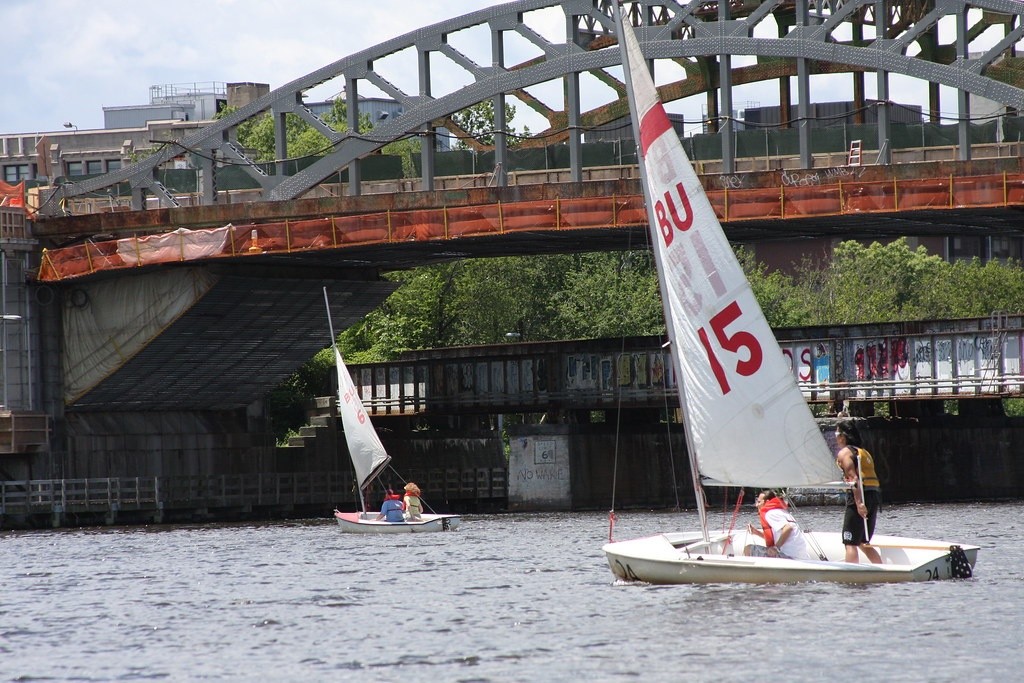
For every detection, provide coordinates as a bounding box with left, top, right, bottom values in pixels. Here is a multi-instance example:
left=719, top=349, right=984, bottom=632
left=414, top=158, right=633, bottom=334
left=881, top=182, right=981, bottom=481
left=375, top=489, right=405, bottom=522
left=402, top=483, right=423, bottom=521
left=743, top=491, right=810, bottom=560
left=834, top=419, right=883, bottom=565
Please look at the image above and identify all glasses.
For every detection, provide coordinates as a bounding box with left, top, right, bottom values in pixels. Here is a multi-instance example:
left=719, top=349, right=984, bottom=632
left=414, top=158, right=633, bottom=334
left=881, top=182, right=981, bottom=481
left=835, top=431, right=848, bottom=437
left=755, top=498, right=763, bottom=504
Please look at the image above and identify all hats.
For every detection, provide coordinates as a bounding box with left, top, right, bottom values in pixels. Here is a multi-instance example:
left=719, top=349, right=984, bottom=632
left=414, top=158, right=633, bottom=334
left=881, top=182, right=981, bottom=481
left=404, top=483, right=420, bottom=492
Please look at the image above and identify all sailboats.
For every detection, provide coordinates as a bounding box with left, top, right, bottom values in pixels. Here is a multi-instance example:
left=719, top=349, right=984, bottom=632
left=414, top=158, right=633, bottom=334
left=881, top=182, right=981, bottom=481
left=322, top=286, right=462, bottom=532
left=603, top=5, right=980, bottom=583
